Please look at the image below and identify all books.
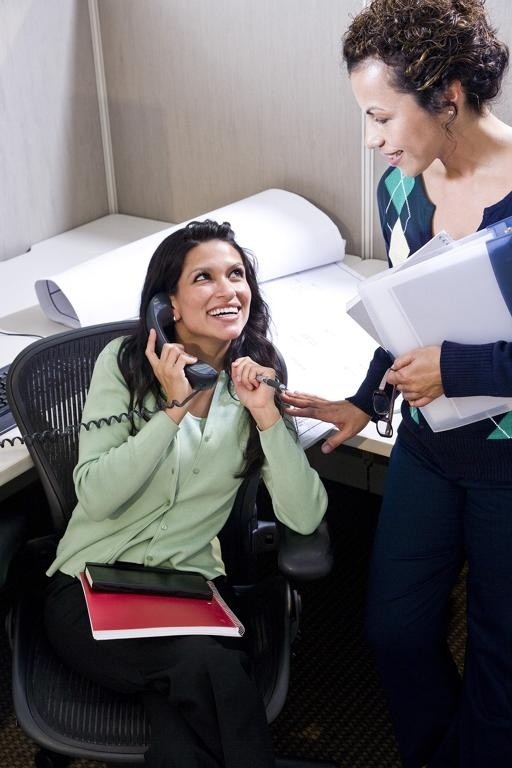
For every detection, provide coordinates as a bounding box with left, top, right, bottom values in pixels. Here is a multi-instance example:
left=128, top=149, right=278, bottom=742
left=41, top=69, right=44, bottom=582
left=83, top=562, right=213, bottom=601
left=345, top=213, right=511, bottom=438
left=75, top=570, right=246, bottom=641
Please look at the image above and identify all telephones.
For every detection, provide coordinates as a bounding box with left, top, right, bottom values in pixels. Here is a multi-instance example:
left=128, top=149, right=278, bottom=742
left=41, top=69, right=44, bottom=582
left=146, top=293, right=218, bottom=391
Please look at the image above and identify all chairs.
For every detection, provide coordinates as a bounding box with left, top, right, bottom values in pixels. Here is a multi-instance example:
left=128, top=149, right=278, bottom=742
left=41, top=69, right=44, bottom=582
left=8, top=318, right=305, bottom=763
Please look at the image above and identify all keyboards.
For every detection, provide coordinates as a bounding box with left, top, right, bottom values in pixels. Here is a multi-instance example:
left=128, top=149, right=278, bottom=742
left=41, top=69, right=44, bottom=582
left=0, top=360, right=92, bottom=432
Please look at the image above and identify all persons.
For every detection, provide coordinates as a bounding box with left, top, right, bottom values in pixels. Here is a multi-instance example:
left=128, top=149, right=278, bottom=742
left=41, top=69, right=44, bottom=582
left=39, top=218, right=334, bottom=768
left=276, top=0, right=508, bottom=767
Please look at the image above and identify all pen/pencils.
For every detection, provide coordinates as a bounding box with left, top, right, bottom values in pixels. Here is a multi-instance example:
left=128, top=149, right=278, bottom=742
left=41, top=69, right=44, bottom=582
left=256, top=374, right=289, bottom=390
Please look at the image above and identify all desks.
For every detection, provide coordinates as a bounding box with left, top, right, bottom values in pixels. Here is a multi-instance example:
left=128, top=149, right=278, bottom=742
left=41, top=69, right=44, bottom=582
left=1, top=212, right=512, bottom=521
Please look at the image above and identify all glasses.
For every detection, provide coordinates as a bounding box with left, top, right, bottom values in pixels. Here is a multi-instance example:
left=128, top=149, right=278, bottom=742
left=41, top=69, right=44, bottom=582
left=372, top=369, right=397, bottom=438
